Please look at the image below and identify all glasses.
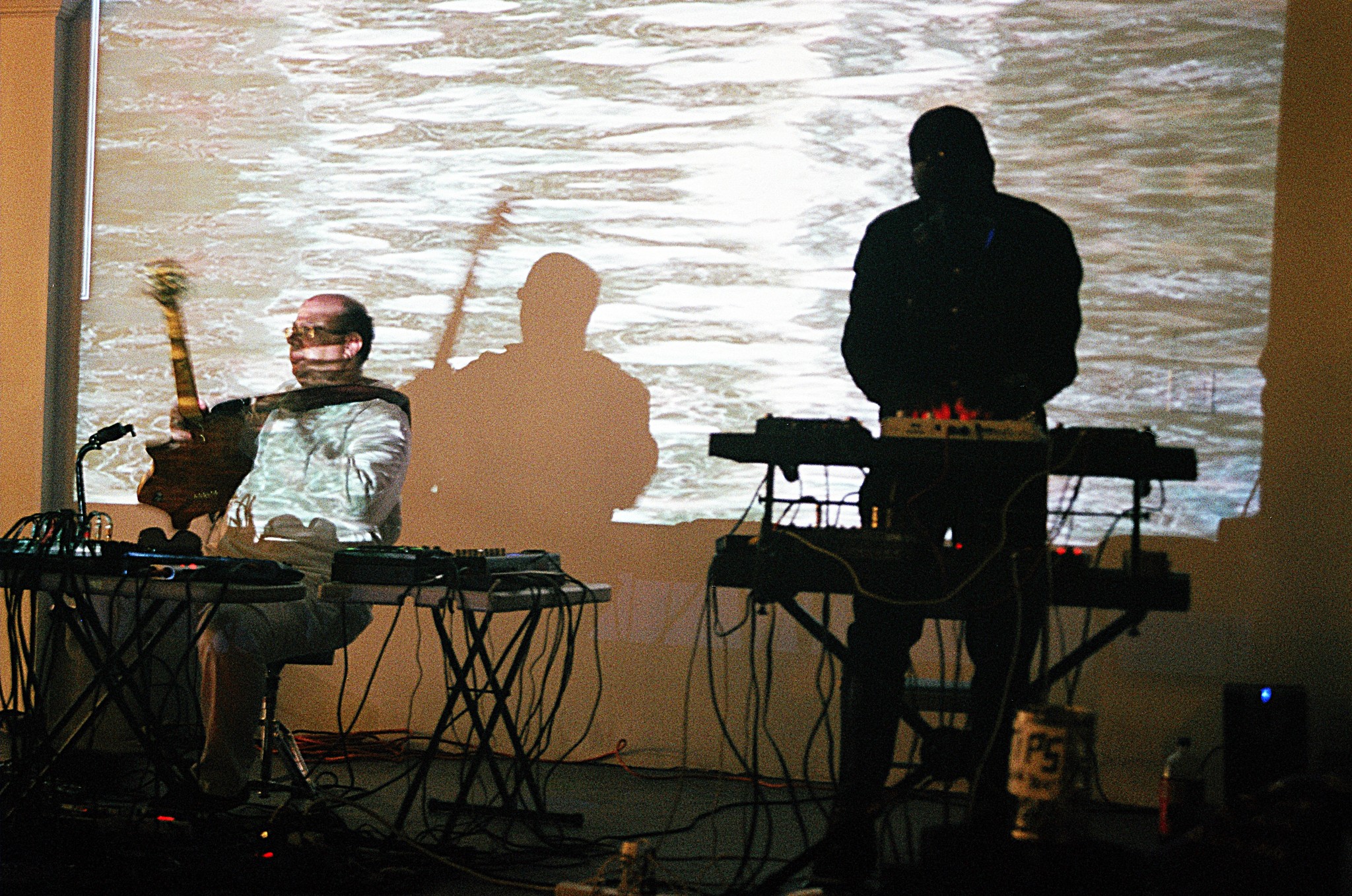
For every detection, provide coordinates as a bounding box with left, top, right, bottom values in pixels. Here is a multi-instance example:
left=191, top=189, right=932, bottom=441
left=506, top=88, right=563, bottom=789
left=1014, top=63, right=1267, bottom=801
left=282, top=325, right=347, bottom=347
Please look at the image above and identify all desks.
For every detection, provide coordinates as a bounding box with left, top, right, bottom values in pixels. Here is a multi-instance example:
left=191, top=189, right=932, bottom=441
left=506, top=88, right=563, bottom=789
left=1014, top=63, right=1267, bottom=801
left=315, top=580, right=614, bottom=866
left=0, top=571, right=311, bottom=838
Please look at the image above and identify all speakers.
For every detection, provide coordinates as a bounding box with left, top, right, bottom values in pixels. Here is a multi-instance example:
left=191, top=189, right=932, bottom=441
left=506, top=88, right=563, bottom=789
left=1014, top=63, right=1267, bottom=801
left=1223, top=683, right=1310, bottom=828
left=50, top=595, right=205, bottom=753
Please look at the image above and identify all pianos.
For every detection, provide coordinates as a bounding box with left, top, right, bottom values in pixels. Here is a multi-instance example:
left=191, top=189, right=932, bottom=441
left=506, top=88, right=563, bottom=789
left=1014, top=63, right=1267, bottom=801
left=710, top=524, right=1194, bottom=895
left=0, top=527, right=310, bottom=896
left=713, top=413, right=1203, bottom=482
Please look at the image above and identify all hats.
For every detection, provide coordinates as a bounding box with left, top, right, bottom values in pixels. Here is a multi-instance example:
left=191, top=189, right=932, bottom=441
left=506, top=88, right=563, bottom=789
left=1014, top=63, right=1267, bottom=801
left=908, top=105, right=986, bottom=163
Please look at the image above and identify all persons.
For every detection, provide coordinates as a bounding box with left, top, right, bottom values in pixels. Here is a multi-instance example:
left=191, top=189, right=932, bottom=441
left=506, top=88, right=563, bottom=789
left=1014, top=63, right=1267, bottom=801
left=146, top=295, right=416, bottom=822
left=799, top=103, right=1082, bottom=896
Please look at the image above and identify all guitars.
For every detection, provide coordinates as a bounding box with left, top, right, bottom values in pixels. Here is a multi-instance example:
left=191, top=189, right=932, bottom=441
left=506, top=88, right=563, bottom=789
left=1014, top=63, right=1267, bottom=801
left=138, top=260, right=249, bottom=525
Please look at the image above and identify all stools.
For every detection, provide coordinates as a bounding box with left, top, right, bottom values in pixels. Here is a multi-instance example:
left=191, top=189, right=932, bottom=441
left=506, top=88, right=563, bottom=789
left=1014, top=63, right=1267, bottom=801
left=252, top=647, right=340, bottom=806
